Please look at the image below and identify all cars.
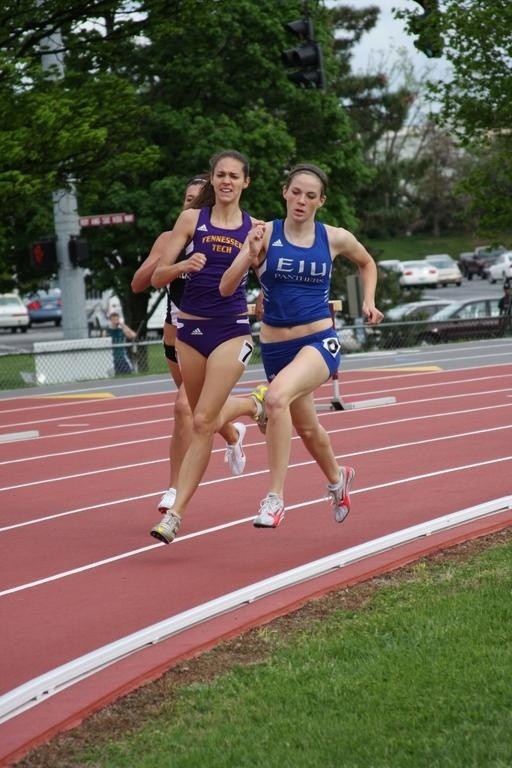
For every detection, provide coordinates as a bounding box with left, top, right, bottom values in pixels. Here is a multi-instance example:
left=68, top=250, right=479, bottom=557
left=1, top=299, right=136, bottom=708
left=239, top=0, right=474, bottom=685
left=328, top=239, right=512, bottom=353
left=0, top=284, right=171, bottom=337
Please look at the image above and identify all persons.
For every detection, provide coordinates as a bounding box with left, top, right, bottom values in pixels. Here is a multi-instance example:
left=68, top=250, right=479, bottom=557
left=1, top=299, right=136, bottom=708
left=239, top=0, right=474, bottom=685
left=220, top=163, right=384, bottom=528
left=131, top=174, right=247, bottom=513
left=150, top=150, right=270, bottom=543
left=101, top=312, right=137, bottom=375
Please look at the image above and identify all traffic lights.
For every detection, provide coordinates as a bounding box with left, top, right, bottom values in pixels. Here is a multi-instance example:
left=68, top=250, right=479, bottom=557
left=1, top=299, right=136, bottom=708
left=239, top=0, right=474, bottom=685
left=30, top=240, right=56, bottom=268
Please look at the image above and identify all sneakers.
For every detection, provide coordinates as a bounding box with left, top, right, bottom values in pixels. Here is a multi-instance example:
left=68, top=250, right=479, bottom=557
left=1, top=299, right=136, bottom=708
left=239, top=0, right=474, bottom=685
left=158, top=487, right=177, bottom=515
left=222, top=421, right=247, bottom=476
left=250, top=384, right=272, bottom=435
left=326, top=466, right=356, bottom=525
left=150, top=510, right=183, bottom=545
left=252, top=492, right=285, bottom=529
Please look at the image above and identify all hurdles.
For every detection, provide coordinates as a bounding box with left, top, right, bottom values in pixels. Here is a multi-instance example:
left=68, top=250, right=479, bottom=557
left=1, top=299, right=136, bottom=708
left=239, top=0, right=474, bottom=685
left=230, top=299, right=396, bottom=412
